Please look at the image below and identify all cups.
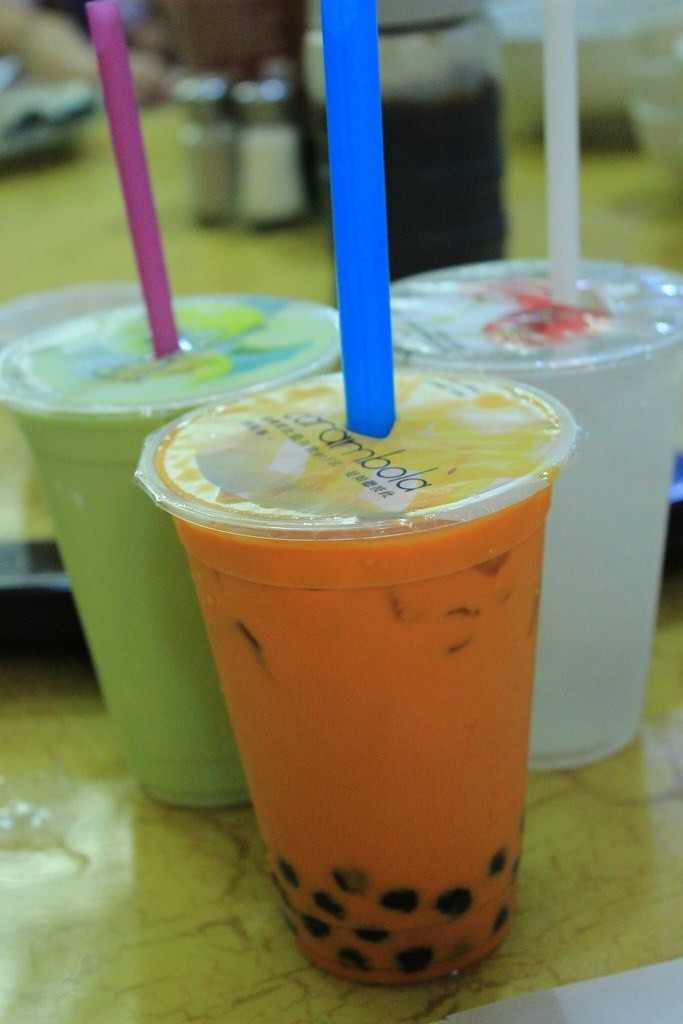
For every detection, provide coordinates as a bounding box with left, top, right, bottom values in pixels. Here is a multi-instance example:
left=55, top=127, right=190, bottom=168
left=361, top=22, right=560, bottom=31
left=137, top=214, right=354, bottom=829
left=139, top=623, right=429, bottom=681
left=135, top=369, right=577, bottom=983
left=0, top=295, right=343, bottom=807
left=390, top=259, right=683, bottom=771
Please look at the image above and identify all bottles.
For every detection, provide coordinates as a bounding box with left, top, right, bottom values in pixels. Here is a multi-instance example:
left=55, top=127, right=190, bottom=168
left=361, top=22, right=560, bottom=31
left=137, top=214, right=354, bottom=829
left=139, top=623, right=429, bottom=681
left=170, top=76, right=237, bottom=227
left=233, top=80, right=312, bottom=228
left=326, top=0, right=507, bottom=278
